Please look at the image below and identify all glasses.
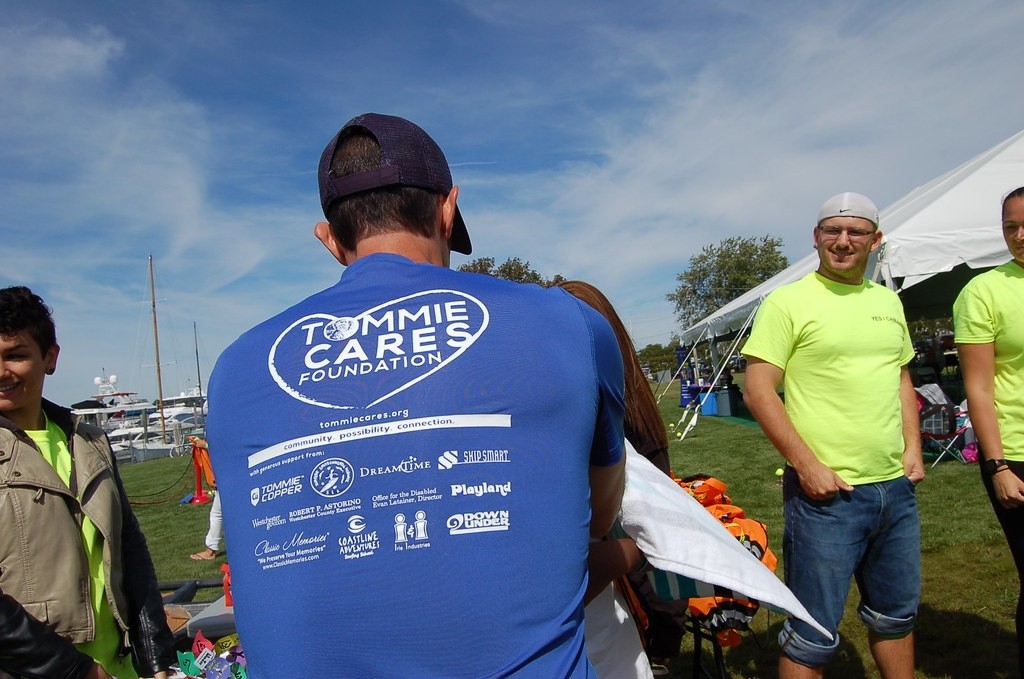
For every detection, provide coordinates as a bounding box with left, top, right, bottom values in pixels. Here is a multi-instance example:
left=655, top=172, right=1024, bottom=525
left=819, top=225, right=874, bottom=241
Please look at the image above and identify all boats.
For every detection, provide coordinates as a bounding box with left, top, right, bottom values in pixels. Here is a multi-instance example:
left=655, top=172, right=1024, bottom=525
left=65, top=365, right=208, bottom=463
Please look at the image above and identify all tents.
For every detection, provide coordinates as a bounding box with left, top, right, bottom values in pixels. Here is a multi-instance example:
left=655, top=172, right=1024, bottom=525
left=655, top=129, right=1024, bottom=387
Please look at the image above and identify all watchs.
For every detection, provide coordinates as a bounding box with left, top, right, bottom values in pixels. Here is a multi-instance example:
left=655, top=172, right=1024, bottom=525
left=983, top=458, right=1006, bottom=475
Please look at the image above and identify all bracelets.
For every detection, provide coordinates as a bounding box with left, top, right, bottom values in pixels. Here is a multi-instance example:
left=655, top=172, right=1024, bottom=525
left=989, top=467, right=1010, bottom=477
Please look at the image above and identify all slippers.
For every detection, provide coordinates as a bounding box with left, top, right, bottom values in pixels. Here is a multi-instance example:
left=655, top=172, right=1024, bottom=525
left=189, top=553, right=208, bottom=562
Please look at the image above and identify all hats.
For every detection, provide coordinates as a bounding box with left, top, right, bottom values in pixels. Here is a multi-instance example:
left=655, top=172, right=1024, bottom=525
left=817, top=192, right=879, bottom=230
left=318, top=113, right=473, bottom=256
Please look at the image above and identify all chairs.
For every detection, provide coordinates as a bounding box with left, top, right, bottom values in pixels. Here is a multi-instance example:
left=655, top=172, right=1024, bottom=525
left=921, top=426, right=968, bottom=468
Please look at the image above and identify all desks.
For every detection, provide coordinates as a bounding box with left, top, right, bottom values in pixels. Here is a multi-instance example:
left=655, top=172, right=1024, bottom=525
left=688, top=385, right=723, bottom=416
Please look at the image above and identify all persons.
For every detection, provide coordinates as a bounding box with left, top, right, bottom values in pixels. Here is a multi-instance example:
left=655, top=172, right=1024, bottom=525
left=0, top=286, right=178, bottom=679
left=190, top=112, right=670, bottom=679
left=740, top=186, right=925, bottom=679
left=953, top=187, right=1024, bottom=679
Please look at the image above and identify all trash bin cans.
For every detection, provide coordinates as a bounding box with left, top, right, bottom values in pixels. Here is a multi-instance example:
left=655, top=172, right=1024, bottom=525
left=715, top=389, right=738, bottom=416
left=699, top=391, right=718, bottom=416
